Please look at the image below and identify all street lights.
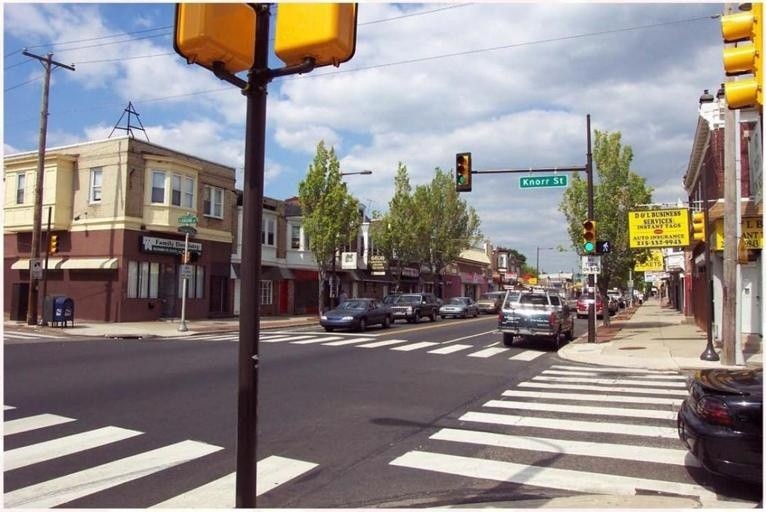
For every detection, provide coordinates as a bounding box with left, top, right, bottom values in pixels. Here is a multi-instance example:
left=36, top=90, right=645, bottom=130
left=536, top=247, right=554, bottom=281
left=330, top=169, right=372, bottom=309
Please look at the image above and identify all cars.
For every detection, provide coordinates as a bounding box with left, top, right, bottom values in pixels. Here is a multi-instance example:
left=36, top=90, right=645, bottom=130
left=575, top=289, right=644, bottom=318
left=319, top=291, right=444, bottom=332
left=438, top=296, right=479, bottom=320
left=675, top=365, right=763, bottom=488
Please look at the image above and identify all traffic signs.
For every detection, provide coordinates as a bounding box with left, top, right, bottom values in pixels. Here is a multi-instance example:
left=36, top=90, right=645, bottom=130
left=177, top=217, right=198, bottom=225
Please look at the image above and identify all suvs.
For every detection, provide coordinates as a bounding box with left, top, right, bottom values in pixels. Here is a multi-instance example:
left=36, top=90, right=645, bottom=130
left=498, top=287, right=574, bottom=351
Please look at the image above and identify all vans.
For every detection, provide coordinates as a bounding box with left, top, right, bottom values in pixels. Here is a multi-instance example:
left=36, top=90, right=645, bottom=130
left=476, top=290, right=507, bottom=314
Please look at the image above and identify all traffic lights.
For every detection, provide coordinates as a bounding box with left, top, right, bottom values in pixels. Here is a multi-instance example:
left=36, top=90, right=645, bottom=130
left=720, top=2, right=763, bottom=110
left=49, top=234, right=60, bottom=255
left=582, top=220, right=596, bottom=254
left=456, top=151, right=471, bottom=192
left=691, top=211, right=706, bottom=244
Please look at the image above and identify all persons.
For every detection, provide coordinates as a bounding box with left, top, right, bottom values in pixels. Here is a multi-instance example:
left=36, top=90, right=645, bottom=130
left=339, top=289, right=349, bottom=302
